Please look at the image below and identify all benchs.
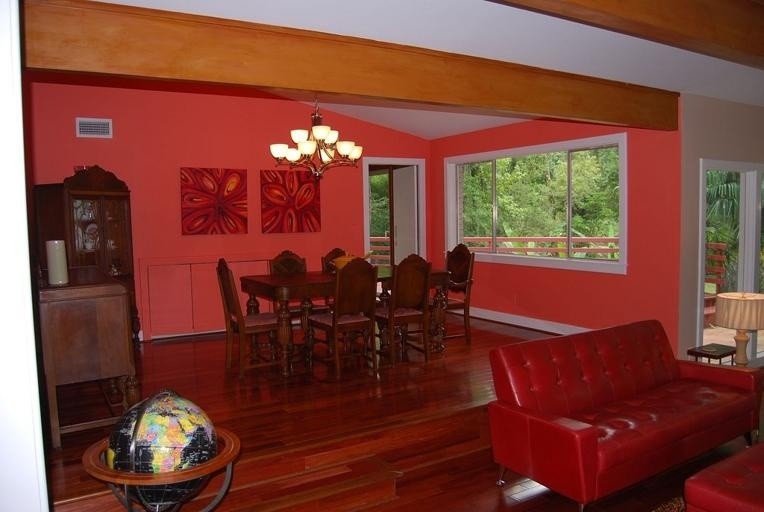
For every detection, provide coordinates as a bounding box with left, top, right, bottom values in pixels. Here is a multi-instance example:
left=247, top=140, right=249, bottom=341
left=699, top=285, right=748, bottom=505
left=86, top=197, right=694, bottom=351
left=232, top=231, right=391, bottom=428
left=486, top=319, right=764, bottom=512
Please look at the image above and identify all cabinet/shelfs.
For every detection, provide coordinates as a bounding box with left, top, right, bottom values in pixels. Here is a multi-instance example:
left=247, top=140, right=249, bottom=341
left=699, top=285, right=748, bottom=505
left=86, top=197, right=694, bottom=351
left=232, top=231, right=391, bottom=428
left=33, top=166, right=141, bottom=352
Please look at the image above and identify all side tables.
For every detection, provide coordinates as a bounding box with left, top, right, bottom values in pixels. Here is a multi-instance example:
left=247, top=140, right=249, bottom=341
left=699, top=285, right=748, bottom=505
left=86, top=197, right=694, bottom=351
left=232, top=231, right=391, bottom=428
left=687, top=341, right=737, bottom=367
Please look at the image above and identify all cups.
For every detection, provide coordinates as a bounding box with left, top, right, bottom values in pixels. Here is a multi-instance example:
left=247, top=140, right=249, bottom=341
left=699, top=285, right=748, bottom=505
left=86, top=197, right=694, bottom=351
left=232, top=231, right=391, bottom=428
left=84, top=240, right=93, bottom=250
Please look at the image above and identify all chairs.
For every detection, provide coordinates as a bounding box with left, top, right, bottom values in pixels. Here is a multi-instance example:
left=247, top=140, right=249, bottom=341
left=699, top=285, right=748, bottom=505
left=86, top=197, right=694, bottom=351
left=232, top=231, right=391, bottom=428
left=217, top=242, right=475, bottom=384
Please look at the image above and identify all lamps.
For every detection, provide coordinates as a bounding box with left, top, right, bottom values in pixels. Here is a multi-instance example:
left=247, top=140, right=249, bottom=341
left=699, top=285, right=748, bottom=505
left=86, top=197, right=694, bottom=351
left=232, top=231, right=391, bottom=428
left=267, top=90, right=364, bottom=181
left=714, top=291, right=764, bottom=367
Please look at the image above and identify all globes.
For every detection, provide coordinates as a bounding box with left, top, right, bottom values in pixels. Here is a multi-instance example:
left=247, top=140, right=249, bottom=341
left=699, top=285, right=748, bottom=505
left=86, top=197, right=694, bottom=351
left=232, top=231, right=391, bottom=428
left=81, top=388, right=241, bottom=512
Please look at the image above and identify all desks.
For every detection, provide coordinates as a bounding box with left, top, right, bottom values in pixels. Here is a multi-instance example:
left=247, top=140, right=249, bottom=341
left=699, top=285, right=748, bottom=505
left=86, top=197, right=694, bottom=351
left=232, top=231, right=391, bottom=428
left=37, top=266, right=142, bottom=449
